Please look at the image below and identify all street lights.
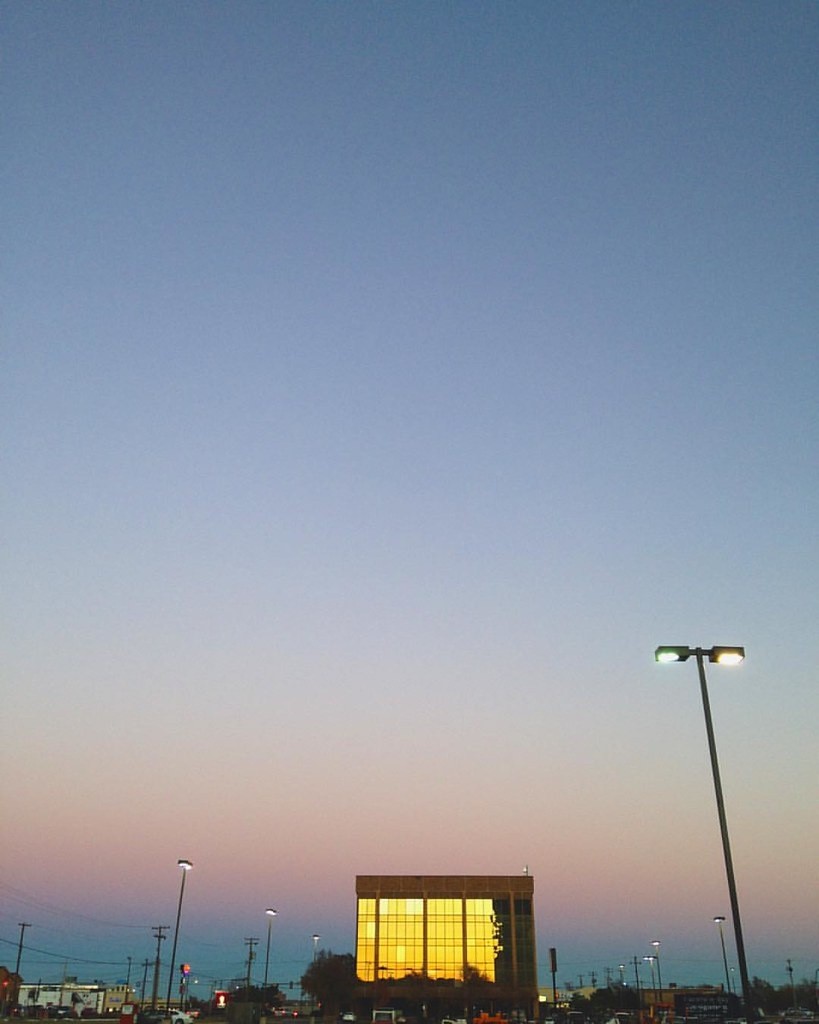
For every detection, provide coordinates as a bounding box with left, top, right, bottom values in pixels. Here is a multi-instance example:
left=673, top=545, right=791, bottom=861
left=312, top=935, right=320, bottom=962
left=653, top=643, right=759, bottom=1024
left=165, top=858, right=192, bottom=1017
left=620, top=964, right=627, bottom=989
left=711, top=916, right=738, bottom=1024
left=644, top=940, right=665, bottom=1004
left=126, top=956, right=132, bottom=1002
left=263, top=909, right=277, bottom=1015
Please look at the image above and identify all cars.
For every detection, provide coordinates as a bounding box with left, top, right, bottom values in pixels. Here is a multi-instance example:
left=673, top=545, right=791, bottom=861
left=186, top=1009, right=201, bottom=1018
left=342, top=1012, right=359, bottom=1022
left=786, top=1008, right=814, bottom=1017
left=142, top=1010, right=194, bottom=1024
left=274, top=1008, right=292, bottom=1016
